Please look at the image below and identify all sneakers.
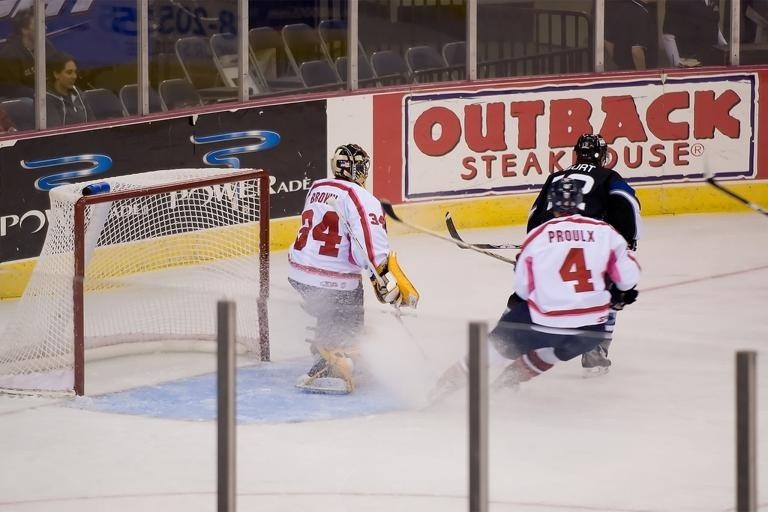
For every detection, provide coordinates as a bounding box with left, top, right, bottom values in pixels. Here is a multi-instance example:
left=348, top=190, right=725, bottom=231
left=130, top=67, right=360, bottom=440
left=581, top=346, right=611, bottom=367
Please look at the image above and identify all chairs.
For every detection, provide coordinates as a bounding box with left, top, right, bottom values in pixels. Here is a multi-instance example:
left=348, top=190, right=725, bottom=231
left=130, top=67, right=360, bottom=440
left=1, top=18, right=467, bottom=137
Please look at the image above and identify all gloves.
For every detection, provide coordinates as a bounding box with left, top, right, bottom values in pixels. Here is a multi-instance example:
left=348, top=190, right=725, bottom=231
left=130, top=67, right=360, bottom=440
left=369, top=265, right=399, bottom=304
left=608, top=281, right=638, bottom=305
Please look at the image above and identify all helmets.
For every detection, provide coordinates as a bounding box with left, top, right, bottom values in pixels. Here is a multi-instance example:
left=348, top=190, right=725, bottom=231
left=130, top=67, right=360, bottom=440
left=547, top=179, right=583, bottom=213
left=573, top=133, right=606, bottom=167
left=331, top=144, right=371, bottom=186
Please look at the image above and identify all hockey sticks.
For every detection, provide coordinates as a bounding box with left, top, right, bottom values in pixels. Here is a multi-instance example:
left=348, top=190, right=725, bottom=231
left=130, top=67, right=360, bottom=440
left=445, top=211, right=523, bottom=250
left=703, top=154, right=768, bottom=216
left=382, top=198, right=518, bottom=265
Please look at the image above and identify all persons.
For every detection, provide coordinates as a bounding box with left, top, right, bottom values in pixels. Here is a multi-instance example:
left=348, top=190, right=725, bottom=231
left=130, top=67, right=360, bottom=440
left=295, top=143, right=419, bottom=393
left=0, top=7, right=95, bottom=99
left=664, top=0, right=731, bottom=69
left=432, top=176, right=642, bottom=408
left=501, top=132, right=640, bottom=378
left=32, top=52, right=98, bottom=128
left=605, top=1, right=655, bottom=71
